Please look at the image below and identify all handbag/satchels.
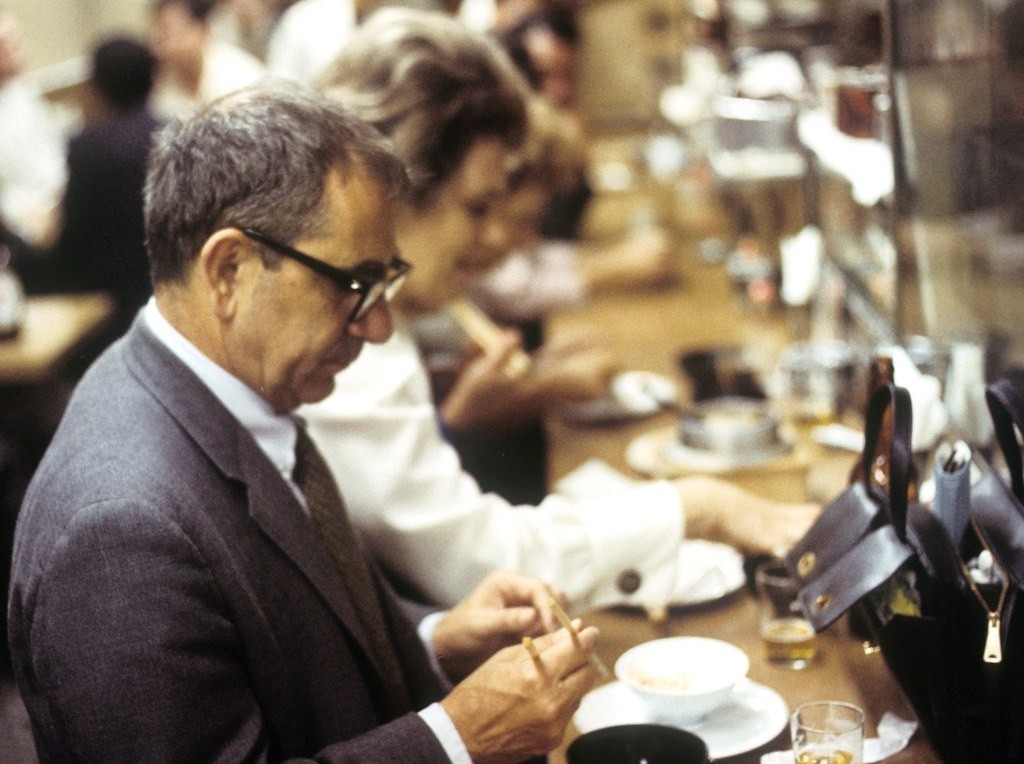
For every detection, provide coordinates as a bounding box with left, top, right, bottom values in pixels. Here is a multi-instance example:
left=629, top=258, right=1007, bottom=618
left=787, top=361, right=1024, bottom=764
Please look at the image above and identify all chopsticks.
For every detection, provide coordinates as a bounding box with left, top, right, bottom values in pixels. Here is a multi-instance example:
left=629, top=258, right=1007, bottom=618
left=526, top=596, right=609, bottom=686
left=452, top=294, right=531, bottom=382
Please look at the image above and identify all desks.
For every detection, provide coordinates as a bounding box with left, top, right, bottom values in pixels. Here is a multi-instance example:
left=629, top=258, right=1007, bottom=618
left=545, top=260, right=938, bottom=764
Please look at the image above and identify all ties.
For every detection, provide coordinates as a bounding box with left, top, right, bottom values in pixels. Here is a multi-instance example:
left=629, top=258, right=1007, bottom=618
left=294, top=419, right=409, bottom=717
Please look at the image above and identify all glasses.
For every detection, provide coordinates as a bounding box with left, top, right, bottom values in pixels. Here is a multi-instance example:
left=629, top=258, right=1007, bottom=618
left=240, top=228, right=412, bottom=325
left=427, top=155, right=533, bottom=221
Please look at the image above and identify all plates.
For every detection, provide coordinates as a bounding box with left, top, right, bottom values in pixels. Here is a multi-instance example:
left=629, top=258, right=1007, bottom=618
left=626, top=424, right=809, bottom=474
left=573, top=680, right=790, bottom=757
left=598, top=529, right=746, bottom=603
left=545, top=371, right=680, bottom=430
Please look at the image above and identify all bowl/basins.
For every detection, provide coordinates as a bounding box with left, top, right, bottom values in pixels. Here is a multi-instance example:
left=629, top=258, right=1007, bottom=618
left=565, top=726, right=707, bottom=764
left=614, top=636, right=749, bottom=721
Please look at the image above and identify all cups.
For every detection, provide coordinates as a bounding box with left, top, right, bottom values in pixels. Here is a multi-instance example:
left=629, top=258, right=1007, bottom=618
left=757, top=560, right=818, bottom=671
left=792, top=701, right=867, bottom=764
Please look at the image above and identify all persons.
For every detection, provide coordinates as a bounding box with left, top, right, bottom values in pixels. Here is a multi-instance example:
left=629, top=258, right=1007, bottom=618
left=7, top=82, right=599, bottom=764
left=0, top=0, right=277, bottom=296
left=264, top=0, right=675, bottom=505
left=295, top=9, right=821, bottom=608
